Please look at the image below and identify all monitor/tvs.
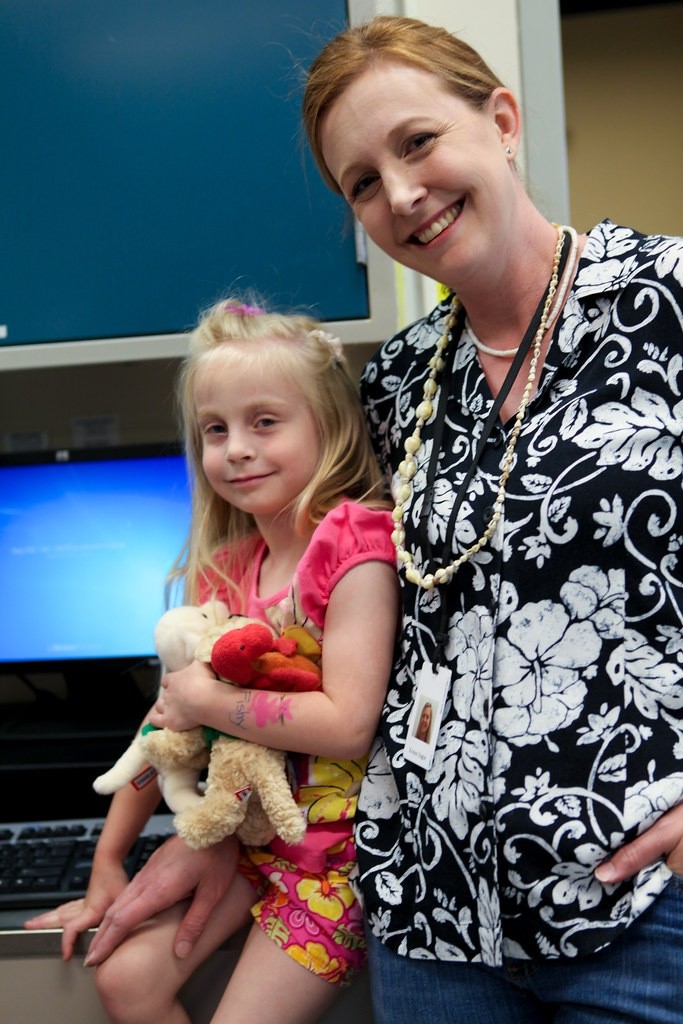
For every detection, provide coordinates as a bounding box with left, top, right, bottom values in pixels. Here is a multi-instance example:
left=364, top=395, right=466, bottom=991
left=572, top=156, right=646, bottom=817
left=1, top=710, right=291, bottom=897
left=0, top=444, right=199, bottom=777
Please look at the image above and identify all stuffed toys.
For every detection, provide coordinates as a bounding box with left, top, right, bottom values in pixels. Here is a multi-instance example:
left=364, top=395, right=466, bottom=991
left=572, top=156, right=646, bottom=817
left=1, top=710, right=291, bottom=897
left=91, top=603, right=325, bottom=850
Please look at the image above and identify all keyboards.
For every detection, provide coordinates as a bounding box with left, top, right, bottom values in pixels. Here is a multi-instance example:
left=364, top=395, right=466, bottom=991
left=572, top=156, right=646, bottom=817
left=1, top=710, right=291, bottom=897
left=0, top=813, right=180, bottom=912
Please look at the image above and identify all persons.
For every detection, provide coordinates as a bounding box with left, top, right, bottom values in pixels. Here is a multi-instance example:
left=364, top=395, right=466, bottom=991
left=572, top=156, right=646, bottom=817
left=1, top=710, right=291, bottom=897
left=26, top=296, right=399, bottom=1022
left=87, top=17, right=682, bottom=1024
left=414, top=703, right=432, bottom=744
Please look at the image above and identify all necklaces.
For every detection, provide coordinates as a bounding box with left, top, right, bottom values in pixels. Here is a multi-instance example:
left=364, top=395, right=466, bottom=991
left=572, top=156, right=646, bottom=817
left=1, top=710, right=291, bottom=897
left=464, top=226, right=578, bottom=357
left=391, top=222, right=565, bottom=583
